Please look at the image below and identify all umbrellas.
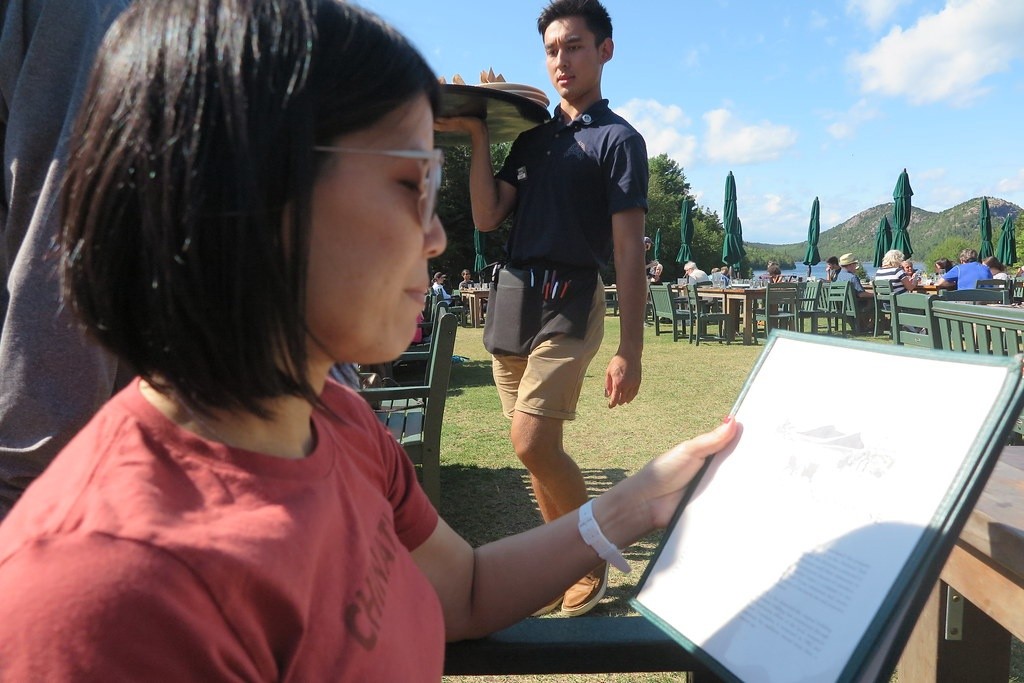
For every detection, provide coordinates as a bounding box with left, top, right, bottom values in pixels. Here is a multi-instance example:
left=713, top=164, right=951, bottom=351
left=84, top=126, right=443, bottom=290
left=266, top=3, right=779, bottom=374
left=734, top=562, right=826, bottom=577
left=996, top=213, right=1017, bottom=267
left=474, top=226, right=490, bottom=324
left=803, top=197, right=822, bottom=277
left=873, top=214, right=892, bottom=267
left=890, top=168, right=913, bottom=261
left=977, top=196, right=994, bottom=264
left=721, top=171, right=746, bottom=281
left=655, top=228, right=663, bottom=263
left=675, top=196, right=694, bottom=283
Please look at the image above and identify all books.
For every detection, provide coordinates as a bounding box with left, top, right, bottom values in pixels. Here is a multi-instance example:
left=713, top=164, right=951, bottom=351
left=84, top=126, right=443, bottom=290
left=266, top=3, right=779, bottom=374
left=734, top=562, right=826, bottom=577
left=625, top=327, right=1024, bottom=683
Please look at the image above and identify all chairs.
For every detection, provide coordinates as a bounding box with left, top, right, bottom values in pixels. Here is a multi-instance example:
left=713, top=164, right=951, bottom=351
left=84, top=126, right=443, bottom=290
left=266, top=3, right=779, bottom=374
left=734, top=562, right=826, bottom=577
left=356, top=289, right=468, bottom=515
left=606, top=276, right=1024, bottom=446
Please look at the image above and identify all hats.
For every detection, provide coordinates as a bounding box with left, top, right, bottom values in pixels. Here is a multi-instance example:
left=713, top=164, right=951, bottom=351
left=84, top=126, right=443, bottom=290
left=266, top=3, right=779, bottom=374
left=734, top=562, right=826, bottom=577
left=434, top=272, right=446, bottom=279
left=839, top=252, right=858, bottom=266
left=645, top=237, right=655, bottom=244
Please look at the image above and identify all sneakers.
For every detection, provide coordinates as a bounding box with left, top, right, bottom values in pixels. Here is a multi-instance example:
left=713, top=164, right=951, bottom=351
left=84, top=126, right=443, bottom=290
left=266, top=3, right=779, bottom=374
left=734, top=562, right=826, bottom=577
left=532, top=561, right=610, bottom=618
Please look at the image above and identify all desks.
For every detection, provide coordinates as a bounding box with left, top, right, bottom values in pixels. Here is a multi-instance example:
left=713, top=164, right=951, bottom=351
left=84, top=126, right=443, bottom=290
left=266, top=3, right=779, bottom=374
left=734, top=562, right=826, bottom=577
left=460, top=290, right=490, bottom=327
left=670, top=284, right=690, bottom=326
left=695, top=287, right=795, bottom=346
left=861, top=284, right=940, bottom=334
left=604, top=286, right=617, bottom=316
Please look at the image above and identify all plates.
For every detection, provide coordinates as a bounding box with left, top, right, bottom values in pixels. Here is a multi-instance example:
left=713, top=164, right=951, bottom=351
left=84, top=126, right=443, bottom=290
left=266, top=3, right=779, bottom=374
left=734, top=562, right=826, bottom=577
left=473, top=82, right=550, bottom=109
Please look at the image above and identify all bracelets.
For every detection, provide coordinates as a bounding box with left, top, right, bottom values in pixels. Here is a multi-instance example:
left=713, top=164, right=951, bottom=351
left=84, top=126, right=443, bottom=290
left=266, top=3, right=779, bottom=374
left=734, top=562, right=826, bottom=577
left=578, top=498, right=632, bottom=574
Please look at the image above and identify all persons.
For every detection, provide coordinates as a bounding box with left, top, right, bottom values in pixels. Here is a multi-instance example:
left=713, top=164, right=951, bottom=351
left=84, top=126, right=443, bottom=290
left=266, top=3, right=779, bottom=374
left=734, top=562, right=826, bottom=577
left=837, top=253, right=878, bottom=311
left=875, top=249, right=923, bottom=333
left=826, top=256, right=842, bottom=283
left=767, top=265, right=790, bottom=288
left=930, top=248, right=1024, bottom=349
left=0, top=0, right=737, bottom=682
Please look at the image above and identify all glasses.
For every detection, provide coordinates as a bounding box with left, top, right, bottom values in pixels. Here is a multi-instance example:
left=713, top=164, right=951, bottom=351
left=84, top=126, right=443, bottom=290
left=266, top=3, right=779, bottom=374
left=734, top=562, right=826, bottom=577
left=311, top=146, right=444, bottom=234
